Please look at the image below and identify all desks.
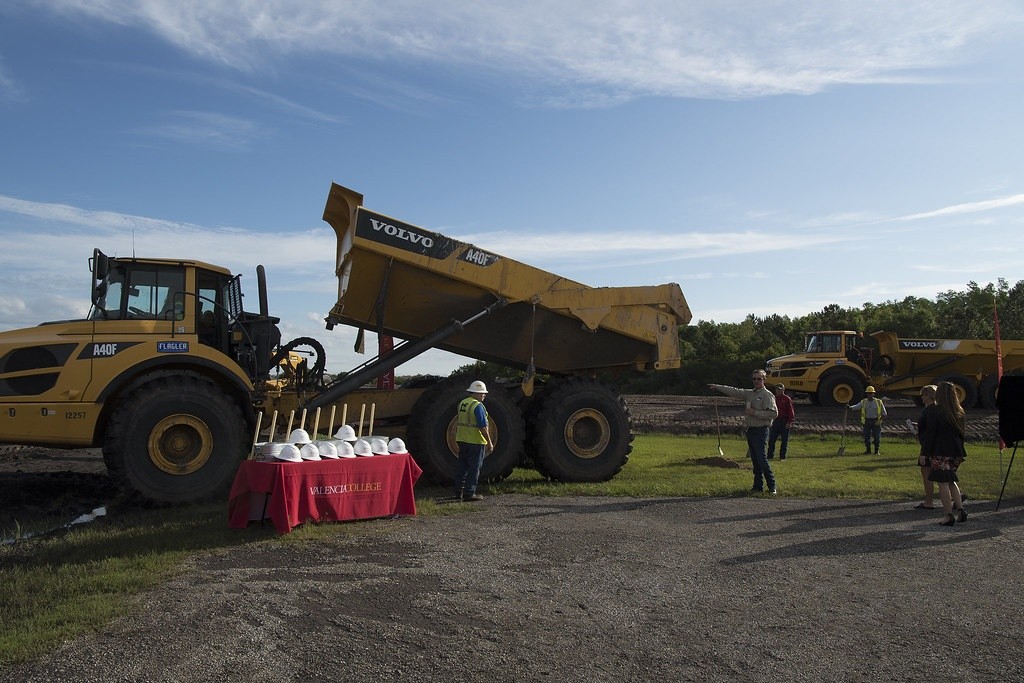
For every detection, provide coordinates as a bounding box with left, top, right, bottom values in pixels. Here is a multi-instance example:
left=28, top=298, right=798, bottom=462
left=226, top=453, right=423, bottom=533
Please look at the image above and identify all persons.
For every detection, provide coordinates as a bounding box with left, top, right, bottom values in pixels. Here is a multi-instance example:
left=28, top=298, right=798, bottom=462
left=908, top=381, right=969, bottom=526
left=455, top=381, right=493, bottom=501
left=706, top=369, right=778, bottom=493
left=847, top=331, right=880, bottom=356
left=845, top=386, right=888, bottom=455
left=766, top=384, right=795, bottom=460
left=808, top=335, right=820, bottom=352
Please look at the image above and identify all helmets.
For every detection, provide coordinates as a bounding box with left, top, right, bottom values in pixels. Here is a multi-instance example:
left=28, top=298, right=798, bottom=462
left=371, top=440, right=390, bottom=455
left=319, top=442, right=339, bottom=458
left=333, top=425, right=358, bottom=442
left=274, top=445, right=304, bottom=463
left=354, top=439, right=375, bottom=456
left=337, top=441, right=358, bottom=459
left=388, top=438, right=408, bottom=454
left=288, top=429, right=313, bottom=444
left=865, top=385, right=876, bottom=393
left=301, top=443, right=322, bottom=460
left=466, top=380, right=489, bottom=394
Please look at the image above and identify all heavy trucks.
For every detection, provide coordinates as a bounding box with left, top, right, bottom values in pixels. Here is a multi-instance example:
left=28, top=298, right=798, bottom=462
left=765, top=329, right=1024, bottom=411
left=0, top=180, right=693, bottom=507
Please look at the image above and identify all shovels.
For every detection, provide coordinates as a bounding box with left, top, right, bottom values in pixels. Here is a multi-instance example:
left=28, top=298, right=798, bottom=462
left=837, top=400, right=849, bottom=456
left=714, top=399, right=724, bottom=455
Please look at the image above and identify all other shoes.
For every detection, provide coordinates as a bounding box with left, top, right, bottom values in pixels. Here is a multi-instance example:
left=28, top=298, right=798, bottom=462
left=746, top=490, right=764, bottom=496
left=770, top=490, right=777, bottom=495
left=466, top=493, right=484, bottom=501
left=457, top=492, right=464, bottom=499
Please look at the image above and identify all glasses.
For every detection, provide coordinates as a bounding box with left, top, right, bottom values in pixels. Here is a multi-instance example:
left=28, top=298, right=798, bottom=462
left=752, top=377, right=764, bottom=381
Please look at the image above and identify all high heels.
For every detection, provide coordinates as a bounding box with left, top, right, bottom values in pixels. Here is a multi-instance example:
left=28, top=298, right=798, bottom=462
left=938, top=514, right=956, bottom=526
left=957, top=509, right=968, bottom=523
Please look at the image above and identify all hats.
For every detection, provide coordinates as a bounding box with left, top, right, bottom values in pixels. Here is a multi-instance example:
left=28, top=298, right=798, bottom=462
left=775, top=383, right=785, bottom=391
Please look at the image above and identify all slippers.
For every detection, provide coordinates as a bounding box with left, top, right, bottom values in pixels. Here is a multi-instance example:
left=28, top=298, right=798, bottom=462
left=914, top=503, right=935, bottom=510
left=952, top=495, right=966, bottom=510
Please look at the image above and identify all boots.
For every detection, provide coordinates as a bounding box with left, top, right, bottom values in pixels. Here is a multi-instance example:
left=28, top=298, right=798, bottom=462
left=874, top=447, right=879, bottom=455
left=864, top=447, right=872, bottom=455
